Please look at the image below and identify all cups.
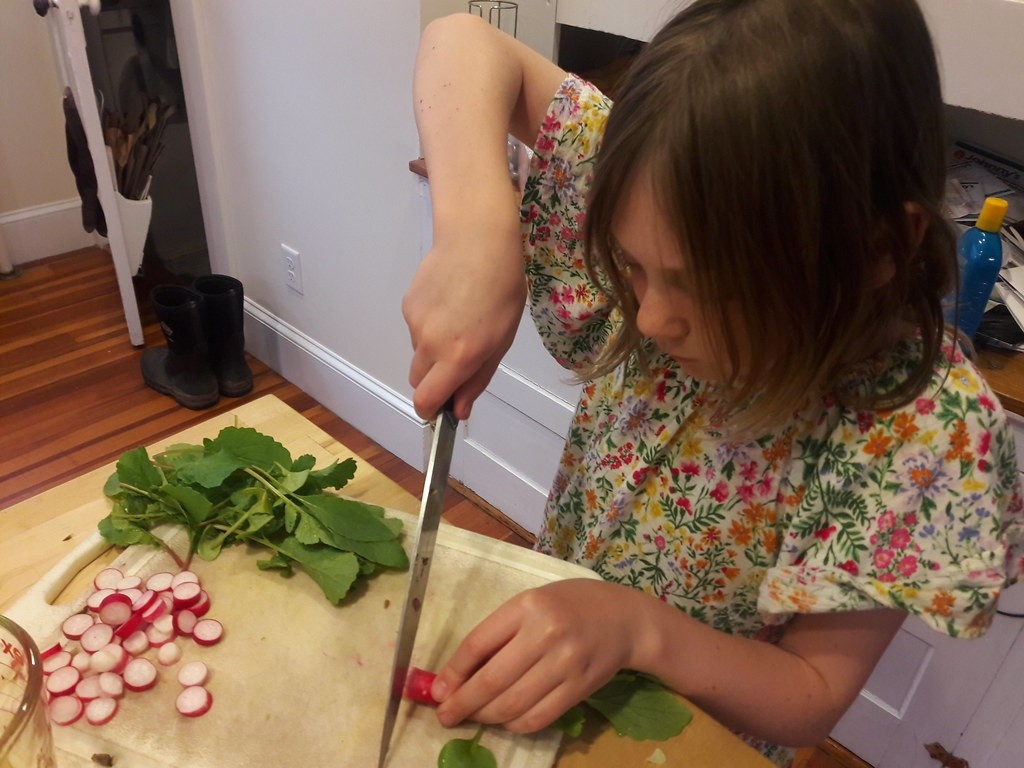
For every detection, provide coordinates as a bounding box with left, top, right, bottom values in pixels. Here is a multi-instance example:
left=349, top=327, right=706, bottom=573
left=0, top=614, right=58, bottom=768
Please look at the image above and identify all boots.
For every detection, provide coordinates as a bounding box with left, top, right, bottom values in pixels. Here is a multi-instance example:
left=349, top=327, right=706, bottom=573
left=140, top=284, right=219, bottom=410
left=193, top=274, right=253, bottom=397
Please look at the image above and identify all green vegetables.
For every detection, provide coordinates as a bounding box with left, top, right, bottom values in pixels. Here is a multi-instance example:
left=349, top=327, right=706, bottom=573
left=96, top=425, right=411, bottom=607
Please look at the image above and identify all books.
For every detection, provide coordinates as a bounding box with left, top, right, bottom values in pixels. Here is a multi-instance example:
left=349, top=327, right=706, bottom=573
left=997, top=219, right=1024, bottom=332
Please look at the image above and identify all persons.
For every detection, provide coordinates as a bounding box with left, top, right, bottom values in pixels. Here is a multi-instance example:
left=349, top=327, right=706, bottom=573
left=401, top=0, right=1024, bottom=767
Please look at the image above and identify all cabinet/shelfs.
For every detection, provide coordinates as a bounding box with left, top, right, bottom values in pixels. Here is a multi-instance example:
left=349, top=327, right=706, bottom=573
left=425, top=179, right=1024, bottom=767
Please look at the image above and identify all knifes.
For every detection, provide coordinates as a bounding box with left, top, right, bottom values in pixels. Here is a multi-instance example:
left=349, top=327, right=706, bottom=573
left=374, top=371, right=461, bottom=768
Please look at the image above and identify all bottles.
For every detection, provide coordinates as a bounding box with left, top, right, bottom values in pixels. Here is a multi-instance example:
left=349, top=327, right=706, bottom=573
left=940, top=197, right=1008, bottom=358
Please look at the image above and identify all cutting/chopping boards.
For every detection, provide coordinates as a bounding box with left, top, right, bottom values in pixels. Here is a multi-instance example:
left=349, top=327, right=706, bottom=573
left=0, top=481, right=606, bottom=768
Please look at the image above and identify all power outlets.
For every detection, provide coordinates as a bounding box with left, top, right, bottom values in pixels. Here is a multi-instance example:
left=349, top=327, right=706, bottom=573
left=282, top=245, right=303, bottom=294
